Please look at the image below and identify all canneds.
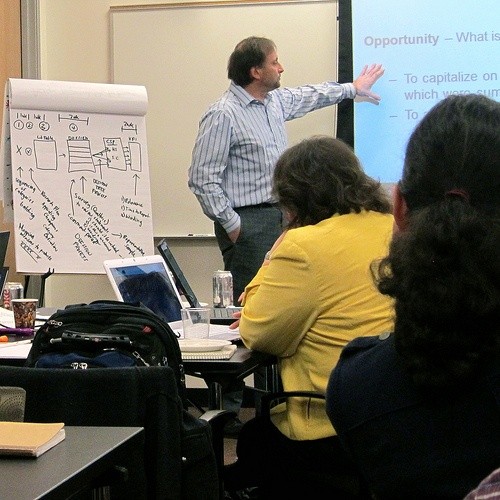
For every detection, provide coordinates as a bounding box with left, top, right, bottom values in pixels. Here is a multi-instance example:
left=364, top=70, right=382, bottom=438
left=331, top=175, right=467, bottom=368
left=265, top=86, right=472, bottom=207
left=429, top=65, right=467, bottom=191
left=3, top=282, right=23, bottom=310
left=212, top=270, right=233, bottom=308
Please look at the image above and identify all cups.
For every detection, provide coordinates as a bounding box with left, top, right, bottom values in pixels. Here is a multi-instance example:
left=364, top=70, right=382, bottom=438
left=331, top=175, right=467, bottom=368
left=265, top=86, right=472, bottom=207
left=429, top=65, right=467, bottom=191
left=180, top=308, right=210, bottom=339
left=11, top=298, right=38, bottom=329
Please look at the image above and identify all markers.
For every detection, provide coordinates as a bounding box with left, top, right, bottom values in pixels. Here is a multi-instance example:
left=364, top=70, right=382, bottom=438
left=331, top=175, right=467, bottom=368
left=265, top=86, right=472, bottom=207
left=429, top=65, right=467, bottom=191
left=188, top=232, right=215, bottom=237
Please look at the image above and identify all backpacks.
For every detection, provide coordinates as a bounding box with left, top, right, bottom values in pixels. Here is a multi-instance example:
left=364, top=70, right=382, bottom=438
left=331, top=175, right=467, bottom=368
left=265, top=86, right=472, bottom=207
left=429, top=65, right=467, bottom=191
left=21, top=299, right=188, bottom=411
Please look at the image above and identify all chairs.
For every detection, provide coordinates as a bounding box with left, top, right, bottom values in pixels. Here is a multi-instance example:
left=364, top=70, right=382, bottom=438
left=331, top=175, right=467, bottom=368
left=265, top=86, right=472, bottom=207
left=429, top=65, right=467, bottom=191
left=237, top=389, right=376, bottom=500
left=0, top=362, right=235, bottom=500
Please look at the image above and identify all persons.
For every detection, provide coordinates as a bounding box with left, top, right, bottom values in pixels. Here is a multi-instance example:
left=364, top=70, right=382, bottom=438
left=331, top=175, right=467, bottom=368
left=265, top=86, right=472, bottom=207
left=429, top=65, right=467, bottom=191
left=189, top=36, right=385, bottom=307
left=227, top=137, right=397, bottom=500
left=325, top=95, right=500, bottom=500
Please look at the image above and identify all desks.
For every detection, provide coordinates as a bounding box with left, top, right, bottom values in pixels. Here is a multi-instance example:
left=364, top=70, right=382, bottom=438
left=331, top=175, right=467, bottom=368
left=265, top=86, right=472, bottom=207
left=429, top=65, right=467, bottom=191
left=0, top=427, right=147, bottom=500
left=0, top=304, right=280, bottom=500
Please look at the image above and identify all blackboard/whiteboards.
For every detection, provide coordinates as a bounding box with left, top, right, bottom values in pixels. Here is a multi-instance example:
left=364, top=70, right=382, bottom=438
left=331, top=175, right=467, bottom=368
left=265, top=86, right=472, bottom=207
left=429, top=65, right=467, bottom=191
left=107, top=2, right=339, bottom=242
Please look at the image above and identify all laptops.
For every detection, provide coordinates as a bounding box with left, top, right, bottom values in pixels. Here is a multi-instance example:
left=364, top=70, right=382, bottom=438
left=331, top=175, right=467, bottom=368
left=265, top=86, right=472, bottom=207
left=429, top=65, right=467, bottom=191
left=157, top=238, right=246, bottom=325
left=103, top=254, right=246, bottom=342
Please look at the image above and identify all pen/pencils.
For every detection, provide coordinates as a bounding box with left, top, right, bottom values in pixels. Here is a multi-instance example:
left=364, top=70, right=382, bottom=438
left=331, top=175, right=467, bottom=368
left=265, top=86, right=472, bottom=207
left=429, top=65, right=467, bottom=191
left=0, top=327, right=33, bottom=334
